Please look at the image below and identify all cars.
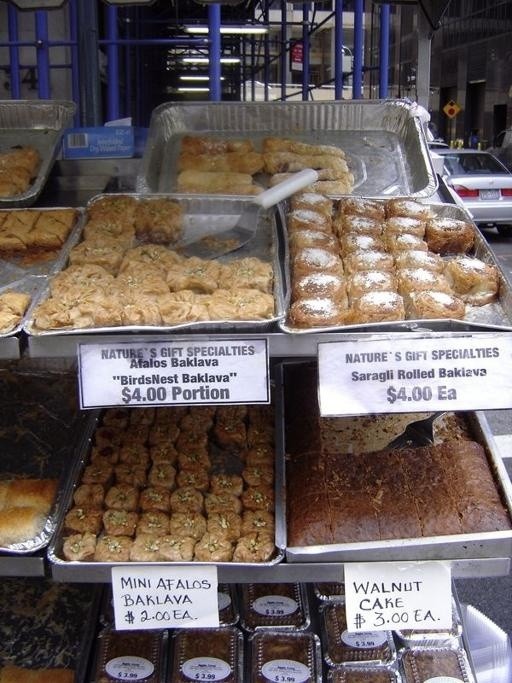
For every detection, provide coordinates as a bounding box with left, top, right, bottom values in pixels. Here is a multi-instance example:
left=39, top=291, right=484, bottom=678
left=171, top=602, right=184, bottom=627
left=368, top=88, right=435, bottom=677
left=430, top=148, right=511, bottom=236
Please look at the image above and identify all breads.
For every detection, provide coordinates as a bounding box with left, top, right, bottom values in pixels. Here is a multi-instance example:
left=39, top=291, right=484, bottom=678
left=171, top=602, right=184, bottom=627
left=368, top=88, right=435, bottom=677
left=92, top=583, right=475, bottom=682
left=1, top=293, right=30, bottom=331
left=0, top=478, right=59, bottom=545
left=285, top=193, right=500, bottom=328
left=34, top=195, right=275, bottom=330
left=0, top=209, right=79, bottom=249
left=62, top=406, right=275, bottom=562
left=283, top=363, right=512, bottom=548
left=0, top=147, right=40, bottom=198
left=0, top=664, right=75, bottom=683
left=177, top=136, right=354, bottom=193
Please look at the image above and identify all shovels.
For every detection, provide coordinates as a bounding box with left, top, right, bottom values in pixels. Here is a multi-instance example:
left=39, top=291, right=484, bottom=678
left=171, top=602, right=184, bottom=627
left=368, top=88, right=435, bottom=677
left=386, top=411, right=449, bottom=450
left=168, top=168, right=319, bottom=260
